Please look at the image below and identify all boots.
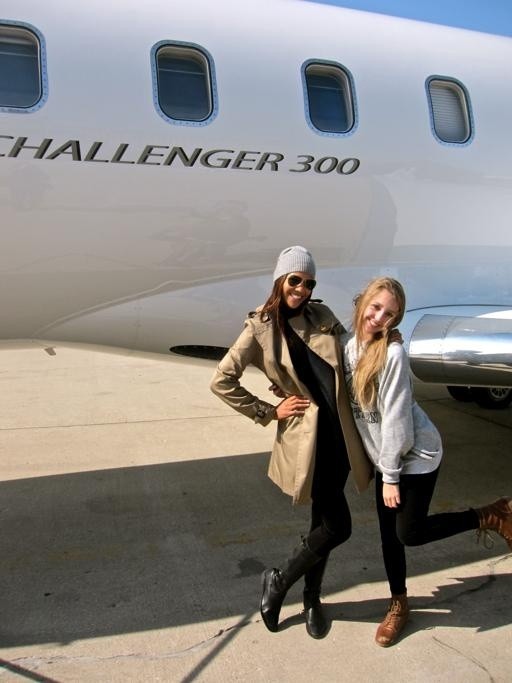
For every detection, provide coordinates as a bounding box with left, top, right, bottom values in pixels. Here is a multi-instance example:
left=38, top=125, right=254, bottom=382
left=303, top=552, right=330, bottom=639
left=375, top=588, right=410, bottom=647
left=259, top=535, right=321, bottom=632
left=477, top=498, right=512, bottom=552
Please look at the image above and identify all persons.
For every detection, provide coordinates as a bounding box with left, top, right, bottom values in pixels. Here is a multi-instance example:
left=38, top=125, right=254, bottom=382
left=210, top=245, right=405, bottom=639
left=268, top=277, right=512, bottom=647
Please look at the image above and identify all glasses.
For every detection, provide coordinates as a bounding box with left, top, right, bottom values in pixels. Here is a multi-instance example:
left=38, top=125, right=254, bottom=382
left=288, top=275, right=316, bottom=290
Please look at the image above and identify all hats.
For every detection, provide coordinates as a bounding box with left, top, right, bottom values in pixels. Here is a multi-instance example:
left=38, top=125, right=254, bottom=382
left=273, top=246, right=316, bottom=286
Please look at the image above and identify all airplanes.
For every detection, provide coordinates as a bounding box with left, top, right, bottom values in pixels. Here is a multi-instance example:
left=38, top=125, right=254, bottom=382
left=1, top=0, right=510, bottom=409
